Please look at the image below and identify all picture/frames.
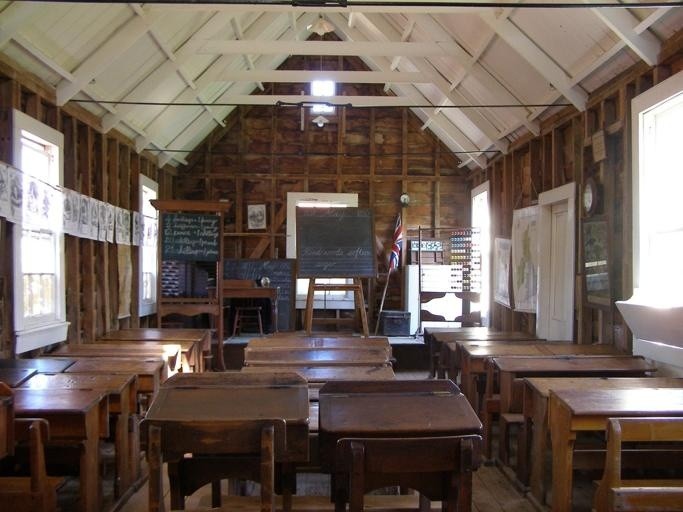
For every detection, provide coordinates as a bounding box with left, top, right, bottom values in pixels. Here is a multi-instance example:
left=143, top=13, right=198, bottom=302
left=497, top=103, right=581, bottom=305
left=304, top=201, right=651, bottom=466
left=246, top=203, right=267, bottom=230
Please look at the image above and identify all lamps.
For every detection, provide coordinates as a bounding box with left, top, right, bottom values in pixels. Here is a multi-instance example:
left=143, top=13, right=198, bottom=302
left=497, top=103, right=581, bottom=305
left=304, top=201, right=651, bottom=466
left=312, top=116, right=330, bottom=128
left=307, top=14, right=336, bottom=36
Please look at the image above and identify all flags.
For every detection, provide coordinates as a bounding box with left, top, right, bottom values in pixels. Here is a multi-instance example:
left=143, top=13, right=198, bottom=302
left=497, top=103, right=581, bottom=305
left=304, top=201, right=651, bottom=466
left=388, top=214, right=404, bottom=272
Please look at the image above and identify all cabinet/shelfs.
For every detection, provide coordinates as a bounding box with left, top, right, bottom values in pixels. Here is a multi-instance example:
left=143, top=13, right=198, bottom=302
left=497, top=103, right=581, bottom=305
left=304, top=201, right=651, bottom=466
left=149, top=199, right=230, bottom=372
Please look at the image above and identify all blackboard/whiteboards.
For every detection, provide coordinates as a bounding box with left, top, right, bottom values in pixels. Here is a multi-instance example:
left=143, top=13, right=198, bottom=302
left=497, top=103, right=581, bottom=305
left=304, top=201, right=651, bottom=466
left=223, top=259, right=295, bottom=332
left=161, top=212, right=220, bottom=262
left=296, top=206, right=378, bottom=279
